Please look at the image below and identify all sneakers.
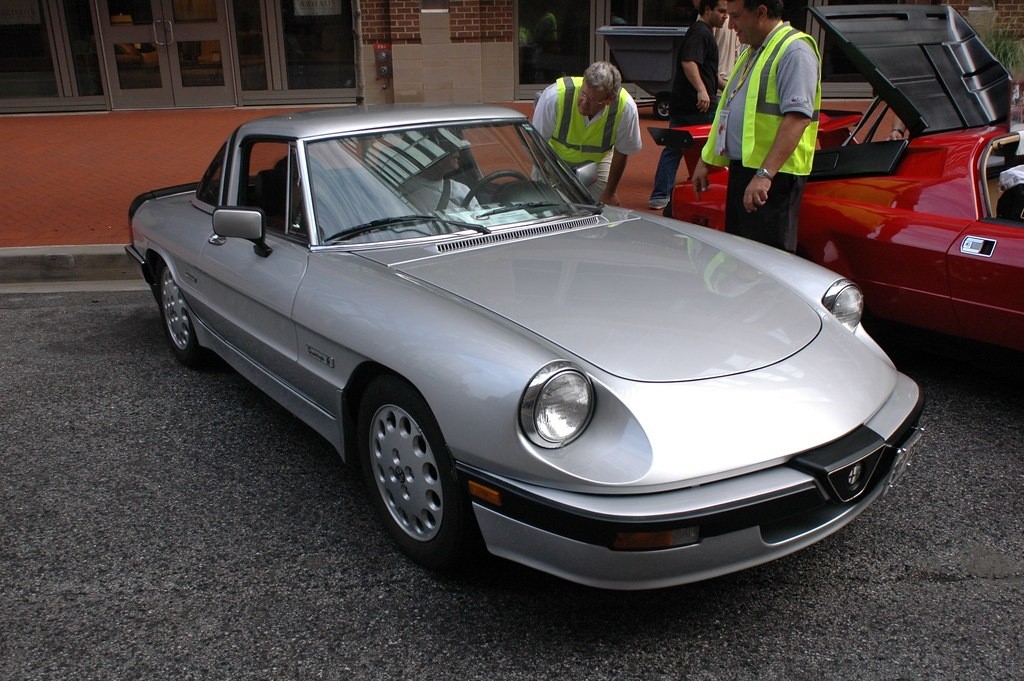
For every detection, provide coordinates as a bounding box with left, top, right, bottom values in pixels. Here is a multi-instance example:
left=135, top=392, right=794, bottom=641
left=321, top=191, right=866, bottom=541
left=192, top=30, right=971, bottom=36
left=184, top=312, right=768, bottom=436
left=649, top=195, right=670, bottom=209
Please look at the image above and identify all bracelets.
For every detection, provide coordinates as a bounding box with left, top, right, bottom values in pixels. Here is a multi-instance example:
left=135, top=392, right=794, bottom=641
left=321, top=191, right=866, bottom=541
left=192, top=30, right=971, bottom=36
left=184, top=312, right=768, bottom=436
left=891, top=129, right=904, bottom=138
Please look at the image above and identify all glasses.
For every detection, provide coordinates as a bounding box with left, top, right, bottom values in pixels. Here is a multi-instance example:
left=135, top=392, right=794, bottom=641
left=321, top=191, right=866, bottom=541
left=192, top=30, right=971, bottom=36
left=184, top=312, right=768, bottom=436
left=577, top=86, right=609, bottom=104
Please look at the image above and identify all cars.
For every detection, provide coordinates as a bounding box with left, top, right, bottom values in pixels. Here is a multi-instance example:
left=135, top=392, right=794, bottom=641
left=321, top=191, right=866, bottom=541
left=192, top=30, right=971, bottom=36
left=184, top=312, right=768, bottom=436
left=122, top=103, right=927, bottom=597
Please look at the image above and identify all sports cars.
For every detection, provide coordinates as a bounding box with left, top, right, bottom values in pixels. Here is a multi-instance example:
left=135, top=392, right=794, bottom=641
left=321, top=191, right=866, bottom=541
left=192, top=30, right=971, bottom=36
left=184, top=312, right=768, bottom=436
left=645, top=1, right=1023, bottom=355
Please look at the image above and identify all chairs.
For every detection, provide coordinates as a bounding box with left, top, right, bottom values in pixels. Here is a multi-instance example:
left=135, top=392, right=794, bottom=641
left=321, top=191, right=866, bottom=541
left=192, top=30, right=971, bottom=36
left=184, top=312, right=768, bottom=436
left=375, top=148, right=405, bottom=188
left=253, top=169, right=279, bottom=218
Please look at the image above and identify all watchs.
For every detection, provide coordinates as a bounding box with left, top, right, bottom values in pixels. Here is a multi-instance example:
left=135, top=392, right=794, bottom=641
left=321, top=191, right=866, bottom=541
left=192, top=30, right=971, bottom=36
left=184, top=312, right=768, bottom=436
left=757, top=168, right=773, bottom=181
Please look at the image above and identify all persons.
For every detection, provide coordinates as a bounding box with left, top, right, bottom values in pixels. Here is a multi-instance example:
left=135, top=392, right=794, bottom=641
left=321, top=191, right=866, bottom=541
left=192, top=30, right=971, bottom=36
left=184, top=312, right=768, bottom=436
left=696, top=13, right=741, bottom=105
left=383, top=139, right=481, bottom=220
left=691, top=0, right=823, bottom=257
left=886, top=116, right=906, bottom=140
left=529, top=61, right=643, bottom=207
left=518, top=7, right=556, bottom=81
left=648, top=0, right=728, bottom=208
